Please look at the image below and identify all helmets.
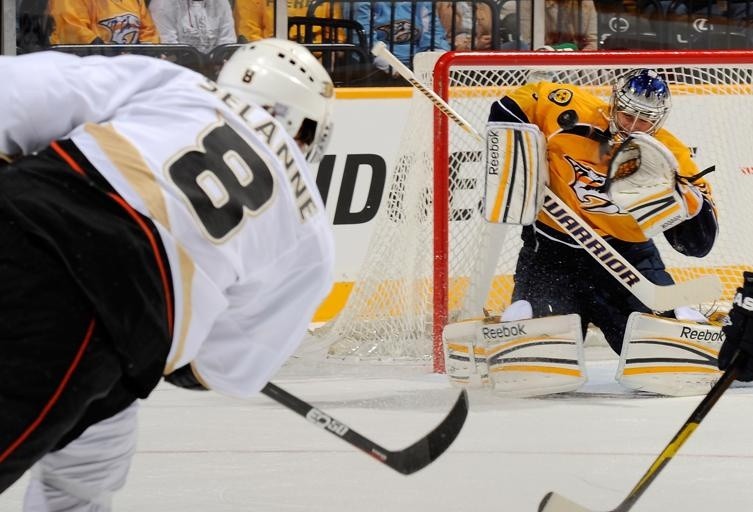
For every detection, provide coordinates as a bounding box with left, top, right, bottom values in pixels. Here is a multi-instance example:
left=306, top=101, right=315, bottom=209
left=213, top=37, right=334, bottom=165
left=610, top=67, right=672, bottom=121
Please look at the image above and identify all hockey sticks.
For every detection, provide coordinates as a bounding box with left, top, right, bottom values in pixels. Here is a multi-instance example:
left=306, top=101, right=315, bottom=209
left=536, top=351, right=750, bottom=512
left=371, top=41, right=722, bottom=312
left=261, top=380, right=469, bottom=475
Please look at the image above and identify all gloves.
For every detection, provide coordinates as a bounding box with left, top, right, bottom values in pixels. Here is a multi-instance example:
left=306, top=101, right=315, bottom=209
left=717, top=271, right=752, bottom=382
